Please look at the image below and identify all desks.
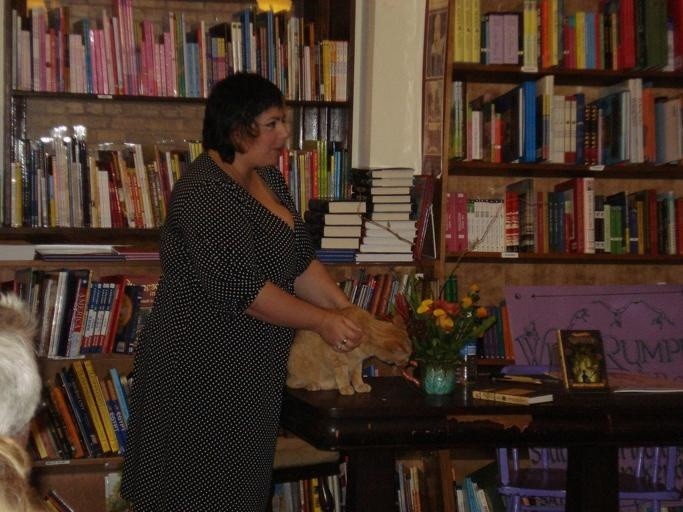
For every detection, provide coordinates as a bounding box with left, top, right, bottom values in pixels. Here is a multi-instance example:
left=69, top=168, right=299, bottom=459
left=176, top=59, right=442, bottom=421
left=279, top=374, right=683, bottom=512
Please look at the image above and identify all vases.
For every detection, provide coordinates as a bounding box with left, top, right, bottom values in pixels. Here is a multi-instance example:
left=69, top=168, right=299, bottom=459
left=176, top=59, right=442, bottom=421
left=424, top=362, right=456, bottom=396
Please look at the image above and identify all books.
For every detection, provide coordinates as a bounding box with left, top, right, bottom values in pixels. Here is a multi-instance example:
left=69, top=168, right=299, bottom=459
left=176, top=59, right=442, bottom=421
left=453, top=75, right=683, bottom=168
left=27, top=360, right=130, bottom=461
left=473, top=385, right=553, bottom=405
left=0, top=268, right=160, bottom=357
left=11, top=137, right=203, bottom=229
left=444, top=176, right=683, bottom=255
left=336, top=274, right=425, bottom=318
left=454, top=0, right=683, bottom=72
left=275, top=461, right=494, bottom=512
left=276, top=139, right=438, bottom=261
left=11, top=0, right=347, bottom=101
left=445, top=275, right=513, bottom=359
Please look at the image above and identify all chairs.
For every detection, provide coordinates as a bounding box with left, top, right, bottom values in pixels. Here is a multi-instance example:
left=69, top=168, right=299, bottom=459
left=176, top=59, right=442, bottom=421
left=494, top=366, right=683, bottom=512
left=267, top=437, right=339, bottom=512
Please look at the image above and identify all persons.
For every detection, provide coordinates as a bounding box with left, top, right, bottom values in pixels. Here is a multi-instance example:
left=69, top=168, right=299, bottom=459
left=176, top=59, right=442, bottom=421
left=119, top=73, right=371, bottom=512
left=0, top=294, right=52, bottom=512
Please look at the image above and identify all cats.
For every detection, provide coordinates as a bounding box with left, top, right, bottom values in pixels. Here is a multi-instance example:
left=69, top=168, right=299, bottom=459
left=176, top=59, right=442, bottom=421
left=284, top=304, right=414, bottom=397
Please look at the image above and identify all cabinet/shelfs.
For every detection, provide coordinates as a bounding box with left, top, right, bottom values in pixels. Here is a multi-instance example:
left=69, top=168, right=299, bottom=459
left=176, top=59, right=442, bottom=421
left=1, top=1, right=356, bottom=234
left=423, top=1, right=683, bottom=264
left=0, top=261, right=427, bottom=512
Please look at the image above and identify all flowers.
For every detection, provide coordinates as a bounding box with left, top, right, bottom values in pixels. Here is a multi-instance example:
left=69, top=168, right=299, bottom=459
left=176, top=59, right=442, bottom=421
left=384, top=276, right=498, bottom=362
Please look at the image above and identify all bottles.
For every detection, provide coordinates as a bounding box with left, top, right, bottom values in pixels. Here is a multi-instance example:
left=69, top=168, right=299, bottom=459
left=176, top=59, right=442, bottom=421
left=460, top=313, right=478, bottom=387
